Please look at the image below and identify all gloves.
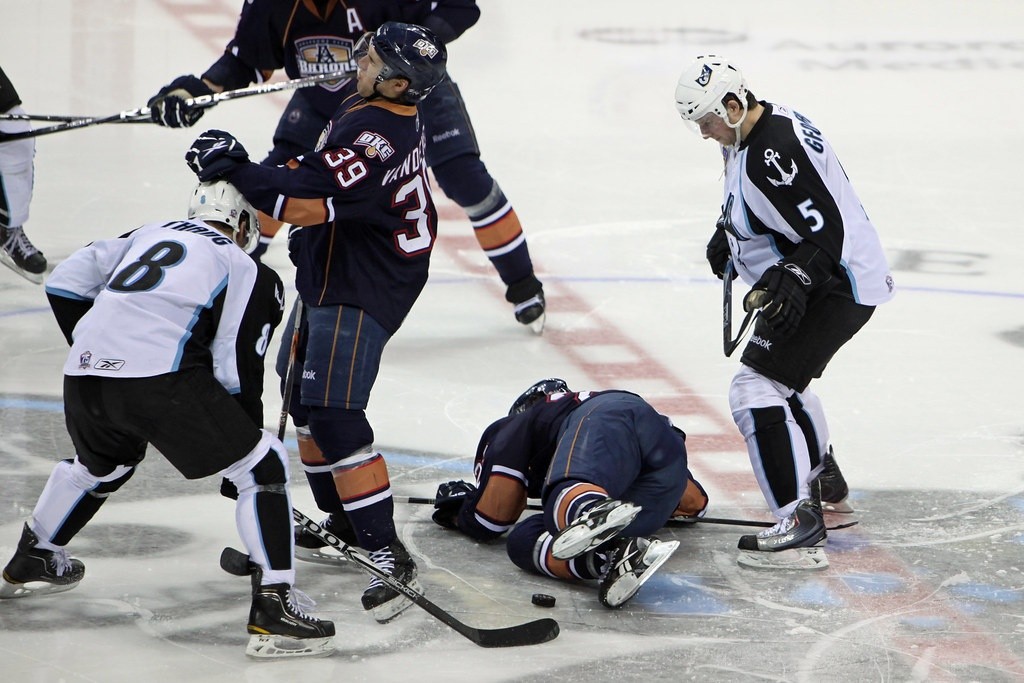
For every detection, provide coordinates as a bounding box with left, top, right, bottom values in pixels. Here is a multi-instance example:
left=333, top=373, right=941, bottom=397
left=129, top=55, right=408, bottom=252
left=437, top=481, right=478, bottom=505
left=146, top=76, right=215, bottom=129
left=187, top=128, right=253, bottom=191
left=742, top=257, right=813, bottom=338
left=706, top=228, right=739, bottom=280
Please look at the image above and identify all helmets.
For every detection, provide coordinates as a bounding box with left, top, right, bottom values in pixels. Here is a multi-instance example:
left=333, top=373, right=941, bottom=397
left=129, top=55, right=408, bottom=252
left=372, top=21, right=447, bottom=105
left=508, top=379, right=569, bottom=417
left=188, top=178, right=260, bottom=254
left=676, top=53, right=748, bottom=128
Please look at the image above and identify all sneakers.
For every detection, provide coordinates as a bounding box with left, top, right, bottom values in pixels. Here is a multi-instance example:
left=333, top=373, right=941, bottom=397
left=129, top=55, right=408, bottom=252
left=550, top=498, right=642, bottom=560
left=293, top=514, right=360, bottom=569
left=511, top=276, right=549, bottom=340
left=0, top=520, right=87, bottom=599
left=246, top=582, right=336, bottom=657
left=0, top=228, right=47, bottom=284
left=813, top=444, right=859, bottom=529
left=361, top=536, right=425, bottom=624
left=735, top=477, right=829, bottom=570
left=597, top=537, right=681, bottom=608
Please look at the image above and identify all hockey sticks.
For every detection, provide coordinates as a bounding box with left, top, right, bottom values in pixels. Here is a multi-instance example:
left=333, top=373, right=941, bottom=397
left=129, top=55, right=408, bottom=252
left=392, top=494, right=778, bottom=528
left=722, top=259, right=763, bottom=358
left=0, top=70, right=357, bottom=147
left=0, top=114, right=162, bottom=124
left=220, top=474, right=561, bottom=648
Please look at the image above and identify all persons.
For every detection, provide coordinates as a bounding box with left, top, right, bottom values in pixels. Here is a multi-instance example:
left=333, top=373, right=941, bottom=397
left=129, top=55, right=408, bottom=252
left=0, top=68, right=48, bottom=285
left=145, top=0, right=549, bottom=335
left=184, top=22, right=449, bottom=625
left=673, top=54, right=894, bottom=571
left=430, top=378, right=708, bottom=608
left=0, top=180, right=337, bottom=659
